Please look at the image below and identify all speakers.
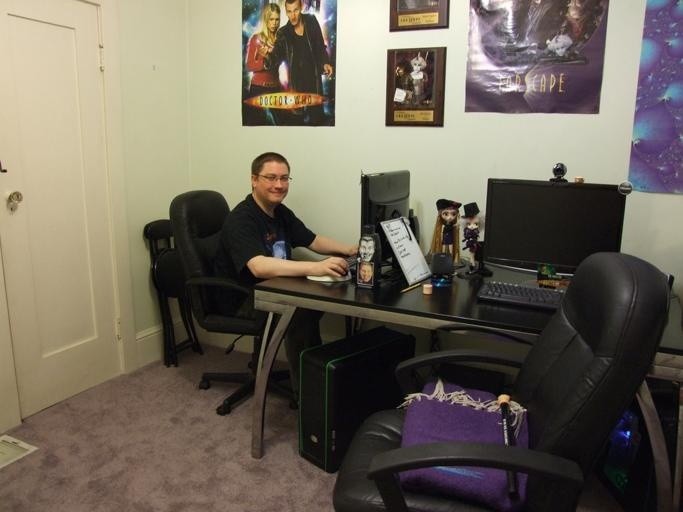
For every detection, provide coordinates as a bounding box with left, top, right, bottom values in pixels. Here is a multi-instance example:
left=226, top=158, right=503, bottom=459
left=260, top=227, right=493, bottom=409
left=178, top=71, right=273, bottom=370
left=431, top=253, right=454, bottom=287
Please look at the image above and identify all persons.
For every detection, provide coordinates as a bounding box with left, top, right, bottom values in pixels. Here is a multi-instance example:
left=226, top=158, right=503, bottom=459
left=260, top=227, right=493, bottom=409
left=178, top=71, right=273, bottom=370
left=359, top=237, right=376, bottom=262
left=216, top=152, right=358, bottom=408
left=358, top=262, right=373, bottom=284
left=461, top=202, right=485, bottom=267
left=270, top=0, right=334, bottom=126
left=431, top=199, right=462, bottom=263
left=245, top=3, right=283, bottom=126
left=537, top=34, right=586, bottom=65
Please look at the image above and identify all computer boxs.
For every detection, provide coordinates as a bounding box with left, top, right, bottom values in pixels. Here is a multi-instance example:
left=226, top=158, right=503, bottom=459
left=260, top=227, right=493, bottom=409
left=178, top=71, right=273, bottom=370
left=606, top=378, right=680, bottom=512
left=297, top=326, right=415, bottom=473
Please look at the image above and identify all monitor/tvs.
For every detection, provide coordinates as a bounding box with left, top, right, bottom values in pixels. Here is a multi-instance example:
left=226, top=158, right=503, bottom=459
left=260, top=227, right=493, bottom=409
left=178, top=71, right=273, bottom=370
left=361, top=169, right=410, bottom=262
left=482, top=178, right=627, bottom=279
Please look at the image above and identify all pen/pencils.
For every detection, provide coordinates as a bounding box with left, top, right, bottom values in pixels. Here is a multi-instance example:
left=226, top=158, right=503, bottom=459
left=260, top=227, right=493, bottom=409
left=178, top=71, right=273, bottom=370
left=400, top=282, right=421, bottom=294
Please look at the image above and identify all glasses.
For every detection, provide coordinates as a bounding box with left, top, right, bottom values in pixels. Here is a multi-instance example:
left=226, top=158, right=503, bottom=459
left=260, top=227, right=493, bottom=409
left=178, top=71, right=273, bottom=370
left=255, top=173, right=293, bottom=186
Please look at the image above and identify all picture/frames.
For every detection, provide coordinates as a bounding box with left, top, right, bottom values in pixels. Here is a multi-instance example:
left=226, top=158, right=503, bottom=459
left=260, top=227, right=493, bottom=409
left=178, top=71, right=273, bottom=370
left=386, top=47, right=447, bottom=127
left=390, top=0, right=449, bottom=32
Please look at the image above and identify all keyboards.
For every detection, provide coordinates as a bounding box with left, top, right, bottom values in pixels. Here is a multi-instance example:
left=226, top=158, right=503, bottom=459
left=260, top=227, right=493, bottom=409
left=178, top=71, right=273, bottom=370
left=345, top=253, right=357, bottom=267
left=476, top=280, right=565, bottom=312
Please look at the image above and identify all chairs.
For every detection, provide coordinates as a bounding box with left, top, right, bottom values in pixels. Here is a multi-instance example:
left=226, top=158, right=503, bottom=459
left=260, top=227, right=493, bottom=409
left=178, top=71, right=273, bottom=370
left=144, top=219, right=203, bottom=367
left=332, top=251, right=671, bottom=512
left=169, top=190, right=325, bottom=416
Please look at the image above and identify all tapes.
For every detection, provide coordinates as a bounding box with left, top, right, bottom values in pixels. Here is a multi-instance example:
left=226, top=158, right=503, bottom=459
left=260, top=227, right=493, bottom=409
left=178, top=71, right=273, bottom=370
left=423, top=284, right=433, bottom=295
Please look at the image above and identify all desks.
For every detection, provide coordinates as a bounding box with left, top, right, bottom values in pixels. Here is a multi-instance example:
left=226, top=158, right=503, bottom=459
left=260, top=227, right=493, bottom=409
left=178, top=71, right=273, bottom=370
left=252, top=255, right=683, bottom=511
